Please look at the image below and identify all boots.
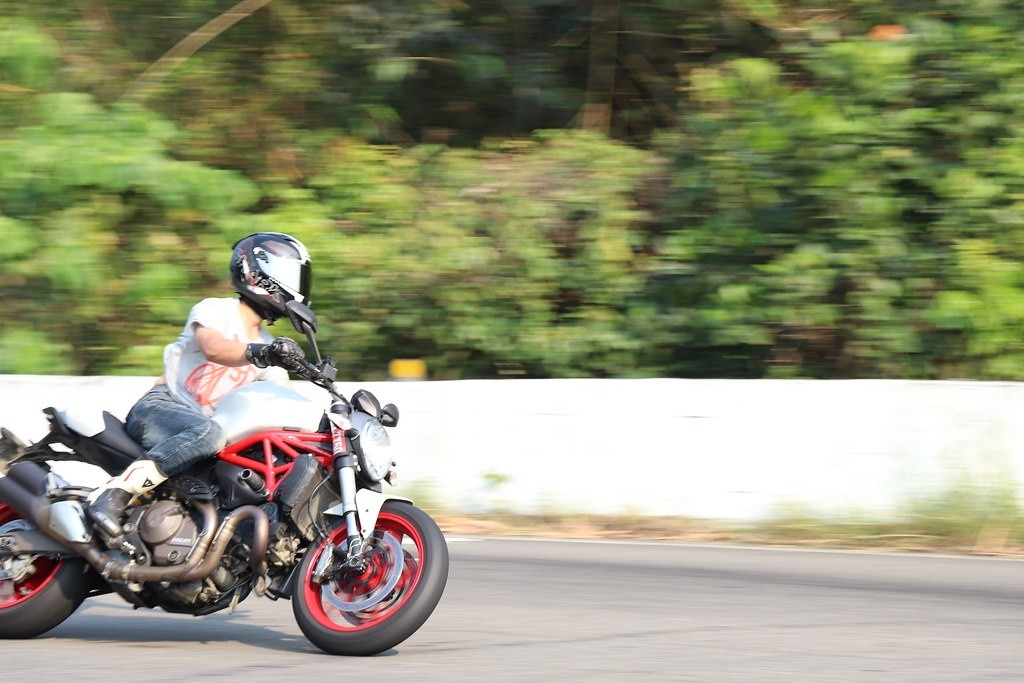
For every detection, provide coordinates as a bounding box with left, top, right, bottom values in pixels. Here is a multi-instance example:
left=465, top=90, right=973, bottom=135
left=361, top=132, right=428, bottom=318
left=86, top=452, right=169, bottom=538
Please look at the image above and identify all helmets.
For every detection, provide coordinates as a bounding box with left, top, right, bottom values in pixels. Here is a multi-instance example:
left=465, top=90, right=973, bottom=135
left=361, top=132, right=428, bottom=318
left=230, top=231, right=311, bottom=316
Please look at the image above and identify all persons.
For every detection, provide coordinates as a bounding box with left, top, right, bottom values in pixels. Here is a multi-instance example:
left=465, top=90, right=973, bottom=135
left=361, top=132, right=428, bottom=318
left=84, top=233, right=311, bottom=537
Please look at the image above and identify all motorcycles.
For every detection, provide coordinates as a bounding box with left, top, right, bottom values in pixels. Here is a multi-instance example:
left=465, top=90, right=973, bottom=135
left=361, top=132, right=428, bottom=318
left=0, top=303, right=449, bottom=656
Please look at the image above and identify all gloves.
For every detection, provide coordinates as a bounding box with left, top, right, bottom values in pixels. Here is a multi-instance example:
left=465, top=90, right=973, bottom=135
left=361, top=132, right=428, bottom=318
left=246, top=336, right=305, bottom=374
